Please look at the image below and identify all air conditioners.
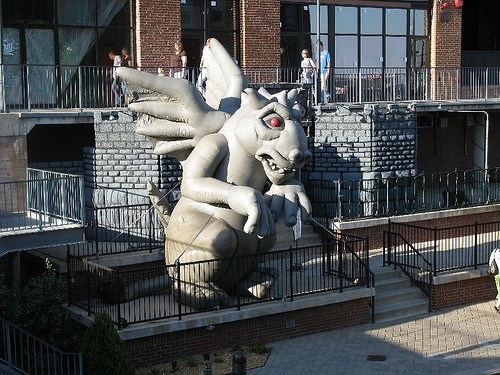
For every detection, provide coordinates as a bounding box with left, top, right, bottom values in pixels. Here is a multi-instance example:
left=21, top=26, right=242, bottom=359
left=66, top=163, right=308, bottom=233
left=416, top=113, right=433, bottom=129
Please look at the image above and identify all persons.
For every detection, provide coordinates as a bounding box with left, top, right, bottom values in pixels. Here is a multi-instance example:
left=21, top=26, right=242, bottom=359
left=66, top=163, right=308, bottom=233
left=108, top=47, right=132, bottom=107
left=300, top=41, right=331, bottom=103
left=489, top=248, right=500, bottom=313
left=169, top=40, right=187, bottom=79
left=195, top=39, right=210, bottom=94
